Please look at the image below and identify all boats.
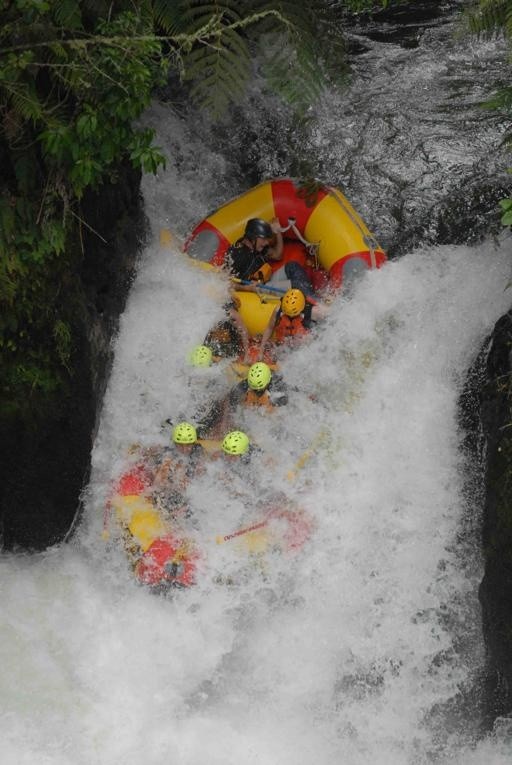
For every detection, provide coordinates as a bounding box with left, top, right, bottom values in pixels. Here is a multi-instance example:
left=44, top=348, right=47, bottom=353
left=98, top=175, right=388, bottom=606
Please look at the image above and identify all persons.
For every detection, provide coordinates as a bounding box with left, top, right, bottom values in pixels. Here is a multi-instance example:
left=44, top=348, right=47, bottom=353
left=204, top=294, right=249, bottom=358
left=223, top=217, right=317, bottom=301
left=257, top=289, right=322, bottom=361
left=230, top=363, right=289, bottom=411
left=209, top=429, right=262, bottom=500
left=173, top=344, right=226, bottom=436
left=153, top=422, right=207, bottom=516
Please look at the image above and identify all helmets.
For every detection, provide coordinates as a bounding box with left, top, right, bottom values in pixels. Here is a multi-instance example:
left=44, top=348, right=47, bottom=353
left=248, top=361, right=272, bottom=391
left=282, top=289, right=305, bottom=318
left=189, top=345, right=213, bottom=369
left=172, top=422, right=198, bottom=445
left=244, top=218, right=274, bottom=240
left=221, top=431, right=250, bottom=456
